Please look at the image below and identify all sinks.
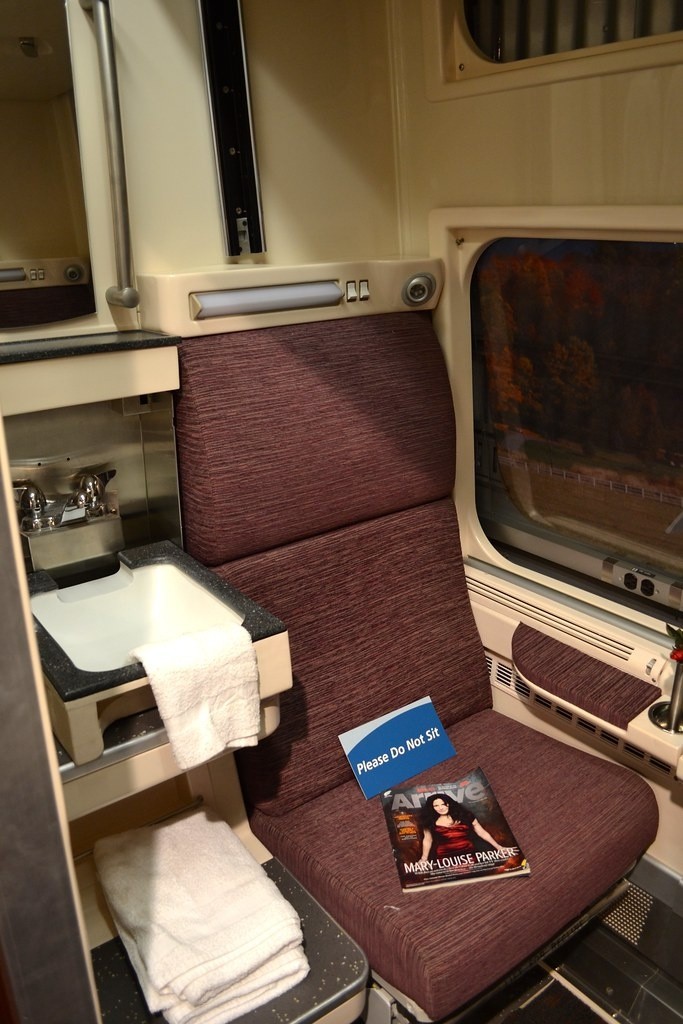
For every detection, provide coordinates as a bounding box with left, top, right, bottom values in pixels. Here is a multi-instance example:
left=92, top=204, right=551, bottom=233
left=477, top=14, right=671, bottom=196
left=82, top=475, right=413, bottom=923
left=30, top=540, right=289, bottom=700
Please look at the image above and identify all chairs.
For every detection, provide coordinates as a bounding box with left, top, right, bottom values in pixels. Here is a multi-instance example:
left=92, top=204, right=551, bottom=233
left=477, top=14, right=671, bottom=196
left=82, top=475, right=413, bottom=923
left=169, top=312, right=661, bottom=1024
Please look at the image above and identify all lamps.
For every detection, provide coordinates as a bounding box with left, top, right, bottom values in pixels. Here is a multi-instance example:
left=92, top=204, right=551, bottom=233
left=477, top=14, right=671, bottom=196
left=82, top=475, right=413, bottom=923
left=188, top=278, right=344, bottom=322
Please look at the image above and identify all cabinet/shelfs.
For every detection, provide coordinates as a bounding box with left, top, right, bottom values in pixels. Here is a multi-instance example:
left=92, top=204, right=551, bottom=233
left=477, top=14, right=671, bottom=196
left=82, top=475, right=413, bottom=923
left=56, top=696, right=372, bottom=1024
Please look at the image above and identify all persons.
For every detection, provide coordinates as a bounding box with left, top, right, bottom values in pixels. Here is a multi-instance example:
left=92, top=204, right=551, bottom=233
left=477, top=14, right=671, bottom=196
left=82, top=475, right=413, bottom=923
left=412, top=793, right=519, bottom=877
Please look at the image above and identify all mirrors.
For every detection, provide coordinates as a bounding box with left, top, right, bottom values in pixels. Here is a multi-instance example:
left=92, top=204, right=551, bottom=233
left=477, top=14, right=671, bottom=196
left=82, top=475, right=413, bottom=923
left=0, top=0, right=91, bottom=293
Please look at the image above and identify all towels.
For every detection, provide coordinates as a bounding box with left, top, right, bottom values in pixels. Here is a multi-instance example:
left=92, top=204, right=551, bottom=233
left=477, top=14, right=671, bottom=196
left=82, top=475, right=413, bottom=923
left=98, top=579, right=265, bottom=774
left=91, top=803, right=312, bottom=1024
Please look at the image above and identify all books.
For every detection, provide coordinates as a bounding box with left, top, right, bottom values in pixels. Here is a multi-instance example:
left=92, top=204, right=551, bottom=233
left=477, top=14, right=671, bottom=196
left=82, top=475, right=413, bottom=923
left=380, top=767, right=532, bottom=894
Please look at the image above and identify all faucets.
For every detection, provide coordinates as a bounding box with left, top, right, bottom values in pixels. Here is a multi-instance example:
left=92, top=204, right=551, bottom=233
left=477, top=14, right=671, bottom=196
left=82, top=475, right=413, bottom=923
left=12, top=466, right=119, bottom=532
